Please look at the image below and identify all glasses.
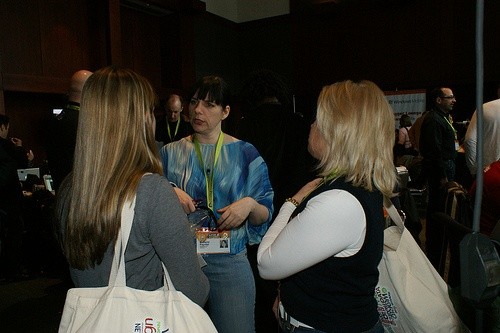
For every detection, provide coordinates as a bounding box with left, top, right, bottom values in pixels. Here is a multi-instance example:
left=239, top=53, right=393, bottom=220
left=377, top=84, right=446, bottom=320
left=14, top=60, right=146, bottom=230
left=440, top=95, right=456, bottom=100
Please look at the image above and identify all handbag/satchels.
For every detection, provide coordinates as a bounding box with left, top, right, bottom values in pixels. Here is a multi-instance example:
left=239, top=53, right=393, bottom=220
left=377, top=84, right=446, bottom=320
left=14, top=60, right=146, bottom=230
left=372, top=190, right=471, bottom=333
left=56, top=171, right=220, bottom=333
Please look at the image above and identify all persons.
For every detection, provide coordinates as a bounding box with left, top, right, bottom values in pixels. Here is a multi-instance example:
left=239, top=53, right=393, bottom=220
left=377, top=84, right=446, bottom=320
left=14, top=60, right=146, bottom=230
left=154, top=92, right=194, bottom=148
left=463, top=158, right=500, bottom=240
left=419, top=86, right=464, bottom=272
left=398, top=114, right=417, bottom=157
left=41, top=69, right=95, bottom=195
left=407, top=105, right=434, bottom=151
left=245, top=71, right=313, bottom=215
left=220, top=240, right=228, bottom=248
left=462, top=94, right=500, bottom=177
left=54, top=64, right=212, bottom=306
left=1, top=114, right=38, bottom=281
left=157, top=74, right=275, bottom=333
left=255, top=81, right=397, bottom=333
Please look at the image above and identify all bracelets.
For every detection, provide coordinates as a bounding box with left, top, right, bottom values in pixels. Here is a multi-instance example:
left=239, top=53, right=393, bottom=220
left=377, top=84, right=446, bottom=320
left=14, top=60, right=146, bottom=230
left=284, top=197, right=298, bottom=207
